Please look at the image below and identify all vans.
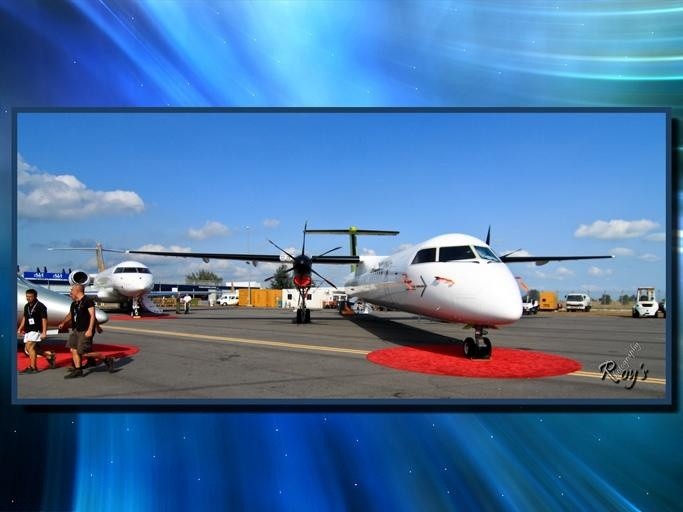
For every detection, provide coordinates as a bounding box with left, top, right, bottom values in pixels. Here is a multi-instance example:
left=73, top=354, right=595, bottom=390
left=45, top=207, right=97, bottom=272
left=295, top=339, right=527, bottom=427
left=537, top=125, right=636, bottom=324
left=217, top=295, right=239, bottom=306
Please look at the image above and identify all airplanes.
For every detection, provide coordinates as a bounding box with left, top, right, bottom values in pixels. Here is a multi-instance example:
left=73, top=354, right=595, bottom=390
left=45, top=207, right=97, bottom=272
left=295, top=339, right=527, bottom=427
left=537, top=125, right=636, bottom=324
left=127, top=222, right=616, bottom=359
left=16, top=243, right=236, bottom=329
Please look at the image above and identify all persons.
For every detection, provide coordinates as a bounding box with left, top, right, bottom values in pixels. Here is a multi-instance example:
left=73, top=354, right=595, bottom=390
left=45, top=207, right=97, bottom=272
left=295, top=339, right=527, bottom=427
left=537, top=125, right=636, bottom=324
left=18, top=288, right=55, bottom=375
left=183, top=293, right=192, bottom=314
left=58, top=284, right=103, bottom=379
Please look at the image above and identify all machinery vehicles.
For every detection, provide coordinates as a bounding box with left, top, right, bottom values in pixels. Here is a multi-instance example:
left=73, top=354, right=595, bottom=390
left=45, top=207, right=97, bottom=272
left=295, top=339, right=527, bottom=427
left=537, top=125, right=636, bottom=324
left=565, top=293, right=592, bottom=312
left=632, top=288, right=659, bottom=319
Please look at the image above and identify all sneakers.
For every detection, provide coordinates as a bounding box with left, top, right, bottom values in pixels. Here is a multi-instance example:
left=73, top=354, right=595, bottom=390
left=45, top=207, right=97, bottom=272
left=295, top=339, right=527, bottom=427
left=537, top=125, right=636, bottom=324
left=104, top=355, right=114, bottom=373
left=63, top=368, right=84, bottom=379
left=46, top=354, right=56, bottom=369
left=19, top=363, right=40, bottom=374
